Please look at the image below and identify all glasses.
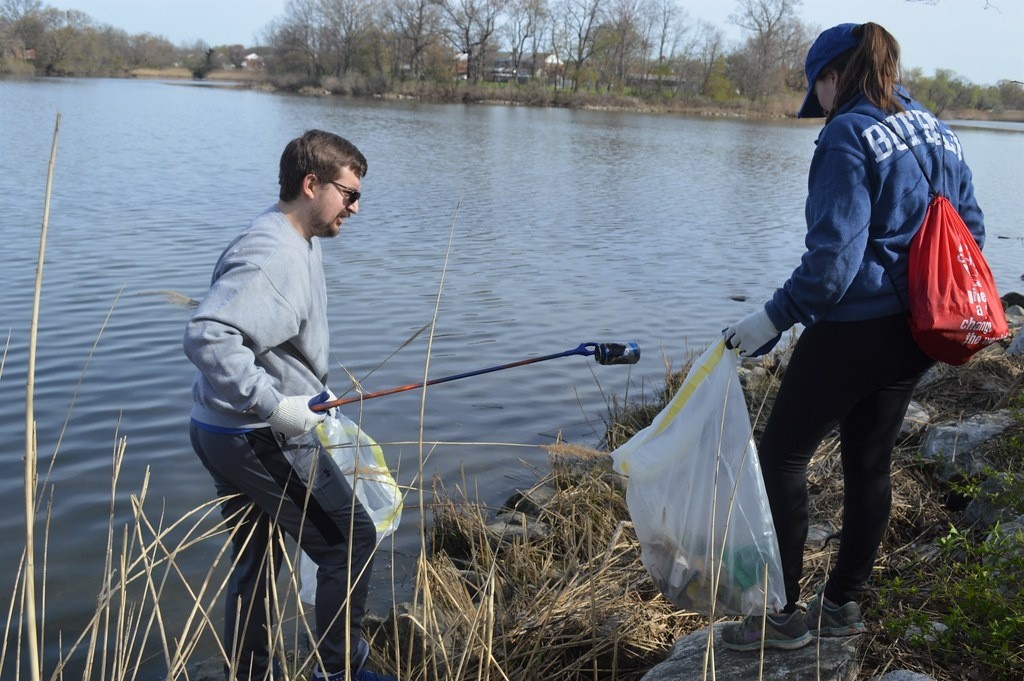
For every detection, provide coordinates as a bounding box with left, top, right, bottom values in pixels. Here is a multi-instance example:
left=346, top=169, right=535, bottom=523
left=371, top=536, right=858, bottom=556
left=320, top=177, right=360, bottom=203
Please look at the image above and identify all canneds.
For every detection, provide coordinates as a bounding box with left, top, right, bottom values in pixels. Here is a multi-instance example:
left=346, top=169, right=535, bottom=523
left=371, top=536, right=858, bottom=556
left=595, top=341, right=642, bottom=365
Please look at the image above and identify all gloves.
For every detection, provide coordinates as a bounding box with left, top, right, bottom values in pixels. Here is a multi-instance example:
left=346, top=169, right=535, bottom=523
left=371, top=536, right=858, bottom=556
left=721, top=308, right=782, bottom=357
left=266, top=389, right=331, bottom=437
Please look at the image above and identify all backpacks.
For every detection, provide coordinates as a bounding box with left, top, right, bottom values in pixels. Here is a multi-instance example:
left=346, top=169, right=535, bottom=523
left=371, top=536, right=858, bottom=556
left=852, top=109, right=1010, bottom=366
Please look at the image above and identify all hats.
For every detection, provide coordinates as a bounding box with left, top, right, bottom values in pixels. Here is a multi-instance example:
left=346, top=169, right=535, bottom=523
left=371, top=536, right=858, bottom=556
left=797, top=23, right=862, bottom=119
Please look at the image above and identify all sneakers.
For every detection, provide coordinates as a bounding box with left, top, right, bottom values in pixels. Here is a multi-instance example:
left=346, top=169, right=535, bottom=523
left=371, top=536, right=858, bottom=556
left=314, top=635, right=396, bottom=681
left=720, top=607, right=813, bottom=651
left=802, top=584, right=866, bottom=636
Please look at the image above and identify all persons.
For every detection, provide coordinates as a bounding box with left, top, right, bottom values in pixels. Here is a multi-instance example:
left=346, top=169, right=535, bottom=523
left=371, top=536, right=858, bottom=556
left=181, top=130, right=393, bottom=681
left=722, top=22, right=984, bottom=649
